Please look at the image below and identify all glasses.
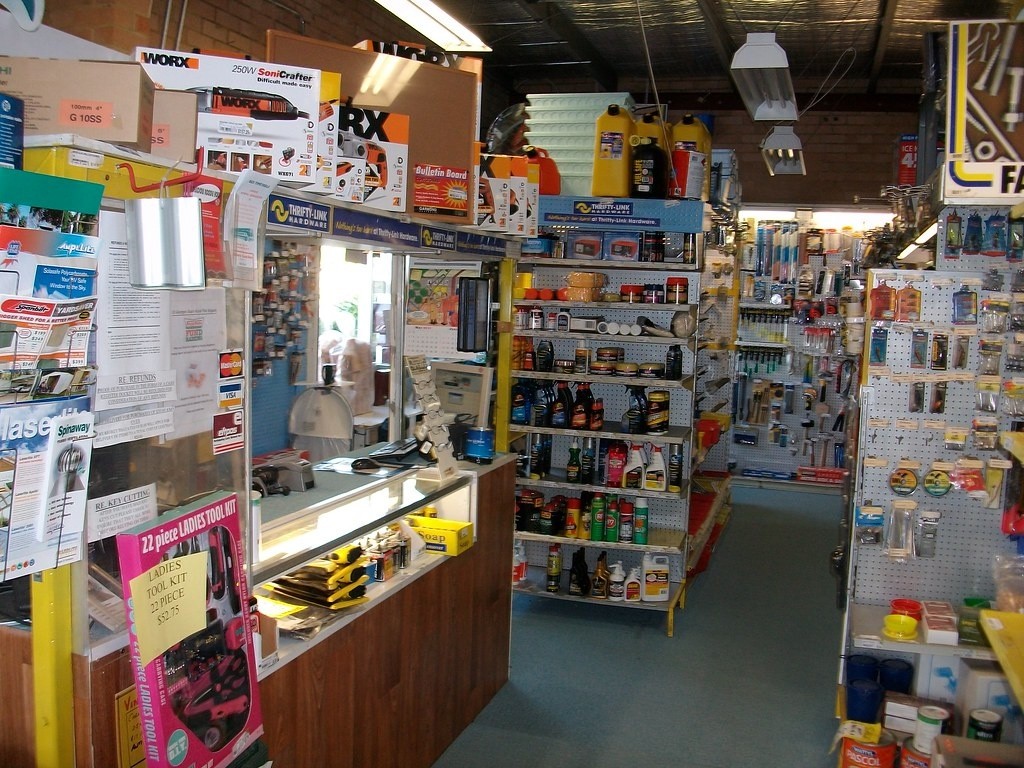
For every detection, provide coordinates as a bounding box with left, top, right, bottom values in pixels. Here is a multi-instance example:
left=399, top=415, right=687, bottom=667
left=434, top=440, right=453, bottom=452
left=423, top=410, right=445, bottom=420
left=427, top=422, right=449, bottom=435
left=414, top=378, right=434, bottom=390
left=413, top=391, right=440, bottom=409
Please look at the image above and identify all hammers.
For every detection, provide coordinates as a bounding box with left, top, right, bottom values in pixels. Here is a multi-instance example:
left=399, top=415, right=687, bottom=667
left=819, top=433, right=838, bottom=470
left=800, top=419, right=816, bottom=456
left=804, top=437, right=818, bottom=466
left=818, top=413, right=831, bottom=432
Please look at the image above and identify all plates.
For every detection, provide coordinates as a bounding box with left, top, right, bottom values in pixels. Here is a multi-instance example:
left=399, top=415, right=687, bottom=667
left=881, top=628, right=918, bottom=639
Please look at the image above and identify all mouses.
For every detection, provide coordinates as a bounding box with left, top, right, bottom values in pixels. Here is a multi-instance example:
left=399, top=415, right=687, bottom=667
left=351, top=459, right=379, bottom=470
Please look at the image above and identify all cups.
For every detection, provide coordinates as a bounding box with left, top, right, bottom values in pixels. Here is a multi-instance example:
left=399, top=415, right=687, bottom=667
left=322, top=364, right=337, bottom=385
left=464, top=427, right=495, bottom=465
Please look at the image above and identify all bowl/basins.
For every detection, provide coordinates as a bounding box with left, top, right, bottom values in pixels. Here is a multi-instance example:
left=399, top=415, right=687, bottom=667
left=884, top=598, right=923, bottom=636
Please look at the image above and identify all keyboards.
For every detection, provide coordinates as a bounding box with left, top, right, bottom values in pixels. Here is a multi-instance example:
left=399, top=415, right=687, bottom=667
left=369, top=437, right=418, bottom=461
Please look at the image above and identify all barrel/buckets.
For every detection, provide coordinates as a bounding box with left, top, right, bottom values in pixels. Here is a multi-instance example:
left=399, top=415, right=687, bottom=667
left=840, top=654, right=1004, bottom=768
left=524, top=145, right=561, bottom=195
left=592, top=103, right=712, bottom=201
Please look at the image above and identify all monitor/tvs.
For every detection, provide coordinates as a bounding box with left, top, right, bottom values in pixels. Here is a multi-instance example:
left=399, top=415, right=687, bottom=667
left=430, top=361, right=495, bottom=429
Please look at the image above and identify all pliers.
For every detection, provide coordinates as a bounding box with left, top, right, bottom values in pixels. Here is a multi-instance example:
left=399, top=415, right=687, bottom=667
left=831, top=405, right=846, bottom=433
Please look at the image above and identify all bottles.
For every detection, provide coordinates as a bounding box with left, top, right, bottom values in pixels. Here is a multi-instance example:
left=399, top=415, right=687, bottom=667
left=252, top=242, right=319, bottom=389
left=509, top=276, right=689, bottom=602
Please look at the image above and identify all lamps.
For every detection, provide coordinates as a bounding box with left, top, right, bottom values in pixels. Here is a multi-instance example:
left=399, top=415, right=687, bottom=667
left=729, top=32, right=805, bottom=177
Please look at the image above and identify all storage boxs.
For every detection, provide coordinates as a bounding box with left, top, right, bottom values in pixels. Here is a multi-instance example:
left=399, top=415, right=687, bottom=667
left=405, top=515, right=475, bottom=557
left=115, top=488, right=259, bottom=765
left=931, top=735, right=1024, bottom=768
left=521, top=92, right=638, bottom=198
left=0, top=45, right=540, bottom=239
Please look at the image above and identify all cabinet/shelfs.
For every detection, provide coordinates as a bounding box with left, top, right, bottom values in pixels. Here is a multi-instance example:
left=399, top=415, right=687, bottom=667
left=223, top=177, right=741, bottom=767
